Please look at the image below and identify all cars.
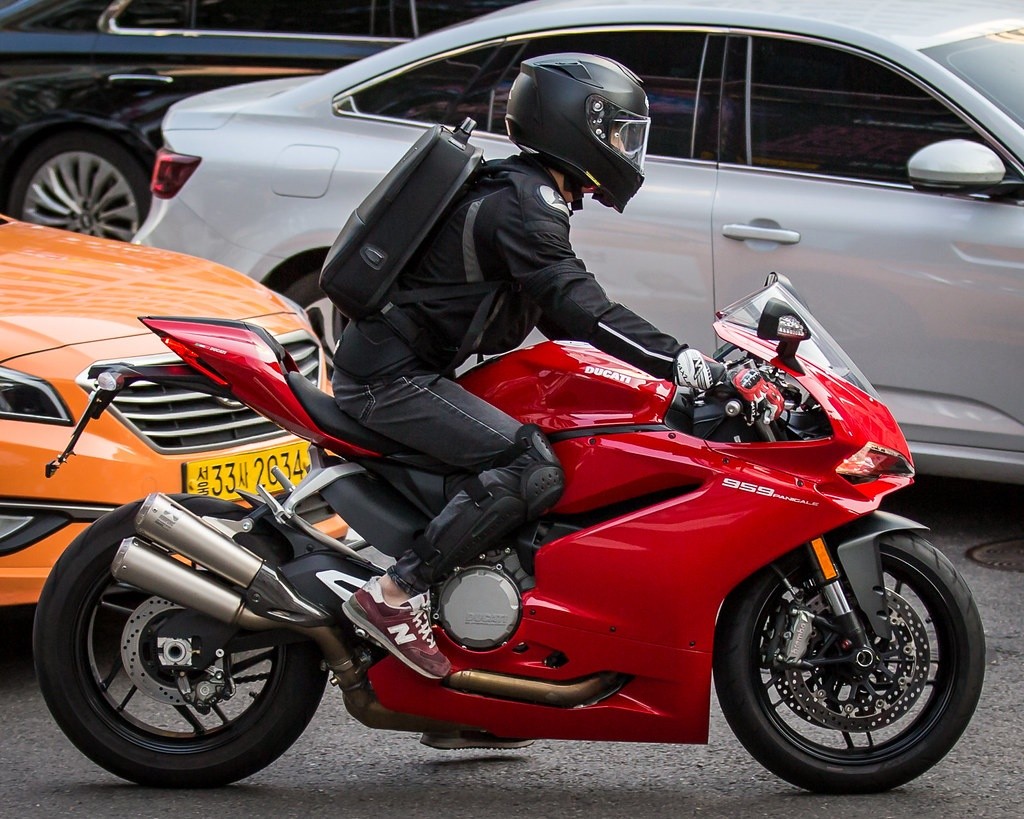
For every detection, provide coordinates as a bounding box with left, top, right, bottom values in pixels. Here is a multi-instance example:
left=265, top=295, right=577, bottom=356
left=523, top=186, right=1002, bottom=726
left=129, top=0, right=1024, bottom=489
left=0, top=209, right=354, bottom=617
left=0, top=0, right=529, bottom=244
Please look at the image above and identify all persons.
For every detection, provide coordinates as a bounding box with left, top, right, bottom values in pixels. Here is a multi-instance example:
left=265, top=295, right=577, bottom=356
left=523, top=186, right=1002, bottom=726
left=331, top=53, right=721, bottom=750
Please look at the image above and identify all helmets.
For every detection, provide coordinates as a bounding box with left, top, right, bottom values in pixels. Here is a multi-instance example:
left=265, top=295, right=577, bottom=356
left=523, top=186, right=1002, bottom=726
left=503, top=53, right=651, bottom=215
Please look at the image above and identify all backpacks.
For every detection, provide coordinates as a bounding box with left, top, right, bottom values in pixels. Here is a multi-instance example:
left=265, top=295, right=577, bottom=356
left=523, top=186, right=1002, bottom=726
left=313, top=114, right=551, bottom=374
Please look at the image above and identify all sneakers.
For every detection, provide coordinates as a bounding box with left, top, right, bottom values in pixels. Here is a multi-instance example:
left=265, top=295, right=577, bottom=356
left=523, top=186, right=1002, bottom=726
left=342, top=575, right=451, bottom=681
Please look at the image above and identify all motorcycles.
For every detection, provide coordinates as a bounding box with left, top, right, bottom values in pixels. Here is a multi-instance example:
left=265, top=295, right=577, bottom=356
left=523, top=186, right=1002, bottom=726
left=27, top=268, right=988, bottom=800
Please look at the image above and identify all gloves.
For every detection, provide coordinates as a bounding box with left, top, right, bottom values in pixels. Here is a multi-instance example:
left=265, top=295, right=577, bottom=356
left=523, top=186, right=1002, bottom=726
left=671, top=348, right=801, bottom=423
